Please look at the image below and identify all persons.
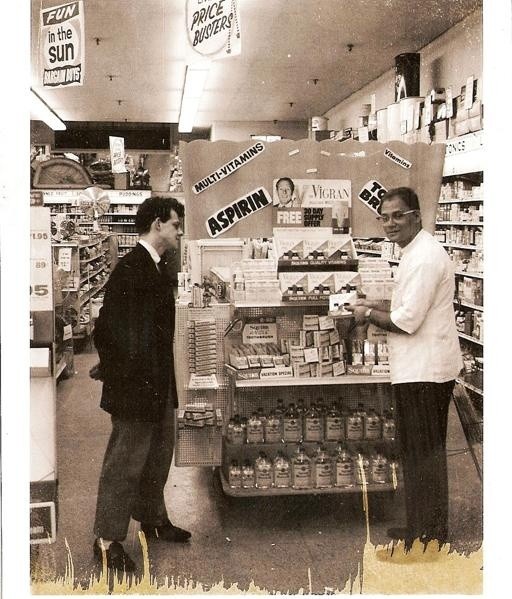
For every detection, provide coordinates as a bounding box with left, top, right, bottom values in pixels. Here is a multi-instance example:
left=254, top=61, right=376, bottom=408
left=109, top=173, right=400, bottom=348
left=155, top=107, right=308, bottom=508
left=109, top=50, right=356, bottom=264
left=342, top=186, right=465, bottom=565
left=272, top=177, right=307, bottom=210
left=88, top=194, right=191, bottom=572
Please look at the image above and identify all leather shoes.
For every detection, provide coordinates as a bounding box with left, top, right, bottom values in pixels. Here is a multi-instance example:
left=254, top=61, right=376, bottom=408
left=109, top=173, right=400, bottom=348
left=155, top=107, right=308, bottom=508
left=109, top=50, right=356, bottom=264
left=94, top=536, right=136, bottom=572
left=141, top=518, right=192, bottom=542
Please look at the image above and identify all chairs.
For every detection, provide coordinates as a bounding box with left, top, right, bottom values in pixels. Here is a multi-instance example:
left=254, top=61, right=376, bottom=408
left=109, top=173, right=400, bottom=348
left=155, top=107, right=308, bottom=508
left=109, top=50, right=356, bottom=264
left=450, top=378, right=482, bottom=481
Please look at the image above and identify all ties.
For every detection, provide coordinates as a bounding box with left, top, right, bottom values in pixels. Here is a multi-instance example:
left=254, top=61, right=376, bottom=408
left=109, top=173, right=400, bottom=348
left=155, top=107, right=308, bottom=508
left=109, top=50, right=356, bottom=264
left=157, top=259, right=167, bottom=288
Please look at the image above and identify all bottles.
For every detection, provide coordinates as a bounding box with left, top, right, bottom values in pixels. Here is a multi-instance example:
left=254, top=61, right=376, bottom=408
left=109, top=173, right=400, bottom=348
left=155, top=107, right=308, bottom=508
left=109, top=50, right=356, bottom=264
left=283, top=251, right=348, bottom=259
left=287, top=286, right=357, bottom=295
left=242, top=235, right=269, bottom=260
left=227, top=397, right=401, bottom=489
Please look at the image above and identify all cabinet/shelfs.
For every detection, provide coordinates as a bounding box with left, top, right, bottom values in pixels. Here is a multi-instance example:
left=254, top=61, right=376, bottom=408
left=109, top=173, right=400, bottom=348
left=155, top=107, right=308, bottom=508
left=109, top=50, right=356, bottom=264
left=48, top=212, right=141, bottom=386
left=209, top=269, right=405, bottom=500
left=433, top=196, right=482, bottom=395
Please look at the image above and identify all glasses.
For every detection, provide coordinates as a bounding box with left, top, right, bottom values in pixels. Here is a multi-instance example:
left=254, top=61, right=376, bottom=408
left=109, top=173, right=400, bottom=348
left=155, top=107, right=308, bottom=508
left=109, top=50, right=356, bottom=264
left=376, top=209, right=416, bottom=224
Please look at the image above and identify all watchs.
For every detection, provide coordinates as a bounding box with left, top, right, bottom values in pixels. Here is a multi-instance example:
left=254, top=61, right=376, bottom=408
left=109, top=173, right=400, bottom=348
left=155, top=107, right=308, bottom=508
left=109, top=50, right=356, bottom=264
left=363, top=307, right=373, bottom=324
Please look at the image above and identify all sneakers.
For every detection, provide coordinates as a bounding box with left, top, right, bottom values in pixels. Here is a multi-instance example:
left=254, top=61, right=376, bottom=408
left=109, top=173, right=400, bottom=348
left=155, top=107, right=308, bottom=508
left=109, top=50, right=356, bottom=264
left=387, top=527, right=447, bottom=553
